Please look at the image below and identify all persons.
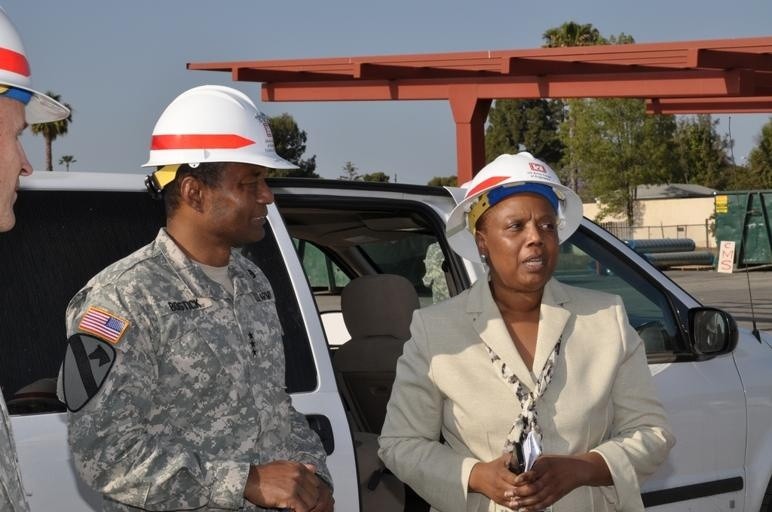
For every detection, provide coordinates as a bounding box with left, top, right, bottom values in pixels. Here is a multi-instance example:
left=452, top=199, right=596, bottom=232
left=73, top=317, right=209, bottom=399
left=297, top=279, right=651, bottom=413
left=63, top=84, right=338, bottom=512
left=378, top=150, right=679, bottom=512
left=0, top=7, right=71, bottom=512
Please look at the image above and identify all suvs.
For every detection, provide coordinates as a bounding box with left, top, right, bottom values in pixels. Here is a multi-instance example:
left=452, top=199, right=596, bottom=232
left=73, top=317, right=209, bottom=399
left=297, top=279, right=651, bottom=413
left=2, top=160, right=772, bottom=510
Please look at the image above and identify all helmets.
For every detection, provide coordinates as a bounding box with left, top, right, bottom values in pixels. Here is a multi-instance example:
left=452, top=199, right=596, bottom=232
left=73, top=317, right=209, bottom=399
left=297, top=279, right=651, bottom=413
left=444, top=150, right=584, bottom=267
left=0, top=7, right=72, bottom=126
left=140, top=84, right=301, bottom=171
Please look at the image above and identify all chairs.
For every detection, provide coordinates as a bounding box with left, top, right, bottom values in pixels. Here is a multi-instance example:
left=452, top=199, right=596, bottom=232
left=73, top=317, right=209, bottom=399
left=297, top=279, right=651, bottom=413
left=333, top=274, right=420, bottom=433
left=353, top=432, right=406, bottom=512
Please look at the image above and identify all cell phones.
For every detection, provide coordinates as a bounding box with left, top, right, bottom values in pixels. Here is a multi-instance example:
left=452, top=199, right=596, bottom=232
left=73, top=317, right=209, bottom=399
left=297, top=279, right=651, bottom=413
left=508, top=444, right=524, bottom=476
